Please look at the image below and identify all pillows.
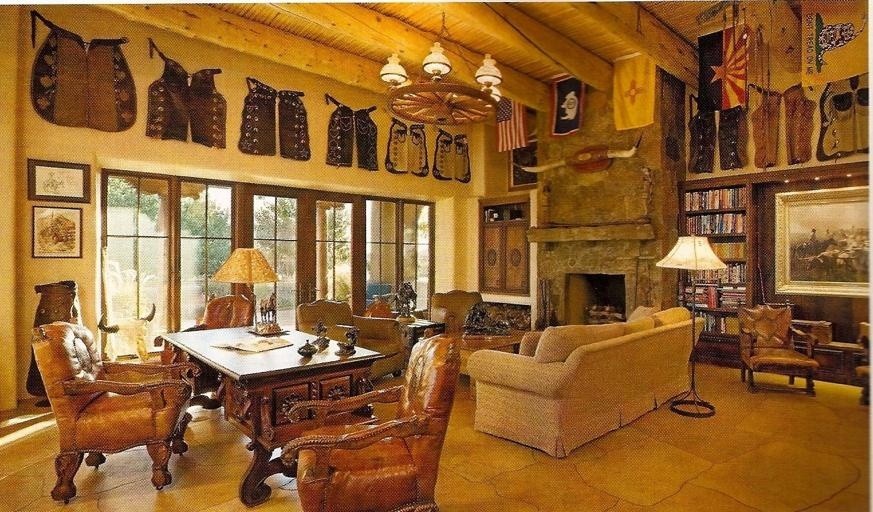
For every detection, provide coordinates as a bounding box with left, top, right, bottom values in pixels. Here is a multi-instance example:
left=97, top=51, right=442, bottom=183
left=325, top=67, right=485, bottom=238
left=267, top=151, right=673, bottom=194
left=520, top=306, right=690, bottom=364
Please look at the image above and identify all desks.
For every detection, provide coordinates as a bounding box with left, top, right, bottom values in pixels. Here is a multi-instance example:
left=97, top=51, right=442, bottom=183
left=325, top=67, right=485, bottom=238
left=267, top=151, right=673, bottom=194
left=160, top=326, right=386, bottom=508
left=401, top=319, right=445, bottom=345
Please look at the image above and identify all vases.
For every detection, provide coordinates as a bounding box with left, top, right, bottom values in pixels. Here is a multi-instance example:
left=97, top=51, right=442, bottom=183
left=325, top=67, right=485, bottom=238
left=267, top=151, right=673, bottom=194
left=678, top=179, right=758, bottom=338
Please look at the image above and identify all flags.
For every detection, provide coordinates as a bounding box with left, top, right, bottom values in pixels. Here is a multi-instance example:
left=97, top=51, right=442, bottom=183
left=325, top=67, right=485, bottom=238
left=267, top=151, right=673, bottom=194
left=496, top=97, right=526, bottom=153
left=802, top=0, right=869, bottom=86
left=549, top=76, right=585, bottom=136
left=698, top=25, right=747, bottom=112
left=612, top=54, right=656, bottom=130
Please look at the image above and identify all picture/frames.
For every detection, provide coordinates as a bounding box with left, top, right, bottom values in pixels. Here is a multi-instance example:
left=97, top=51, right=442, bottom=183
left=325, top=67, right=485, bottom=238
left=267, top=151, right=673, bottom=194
left=774, top=185, right=870, bottom=299
left=28, top=158, right=92, bottom=204
left=32, top=206, right=83, bottom=258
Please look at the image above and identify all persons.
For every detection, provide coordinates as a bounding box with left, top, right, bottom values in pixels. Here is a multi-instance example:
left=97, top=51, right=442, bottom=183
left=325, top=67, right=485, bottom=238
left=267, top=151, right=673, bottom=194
left=803, top=228, right=835, bottom=248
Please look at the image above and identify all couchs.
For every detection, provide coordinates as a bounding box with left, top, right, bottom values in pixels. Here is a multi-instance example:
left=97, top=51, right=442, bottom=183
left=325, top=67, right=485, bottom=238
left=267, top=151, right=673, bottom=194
left=466, top=307, right=706, bottom=458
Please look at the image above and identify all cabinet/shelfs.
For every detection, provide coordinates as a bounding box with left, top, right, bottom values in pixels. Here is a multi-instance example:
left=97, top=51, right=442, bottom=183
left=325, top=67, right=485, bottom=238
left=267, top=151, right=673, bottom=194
left=478, top=194, right=530, bottom=297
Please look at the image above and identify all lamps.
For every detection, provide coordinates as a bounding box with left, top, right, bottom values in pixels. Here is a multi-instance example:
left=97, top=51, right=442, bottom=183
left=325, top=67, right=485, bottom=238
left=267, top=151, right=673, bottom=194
left=212, top=248, right=281, bottom=332
left=380, top=11, right=502, bottom=126
left=656, top=234, right=728, bottom=418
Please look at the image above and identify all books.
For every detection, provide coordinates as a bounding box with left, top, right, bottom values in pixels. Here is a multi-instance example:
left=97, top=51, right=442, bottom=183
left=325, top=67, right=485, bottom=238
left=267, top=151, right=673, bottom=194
left=682, top=189, right=746, bottom=336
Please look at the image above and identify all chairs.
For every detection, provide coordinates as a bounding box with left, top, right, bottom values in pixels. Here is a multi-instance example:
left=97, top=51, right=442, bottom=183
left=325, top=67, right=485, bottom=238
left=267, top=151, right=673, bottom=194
left=297, top=298, right=406, bottom=380
left=162, top=295, right=255, bottom=395
left=739, top=300, right=819, bottom=396
left=431, top=289, right=484, bottom=332
left=32, top=323, right=203, bottom=505
